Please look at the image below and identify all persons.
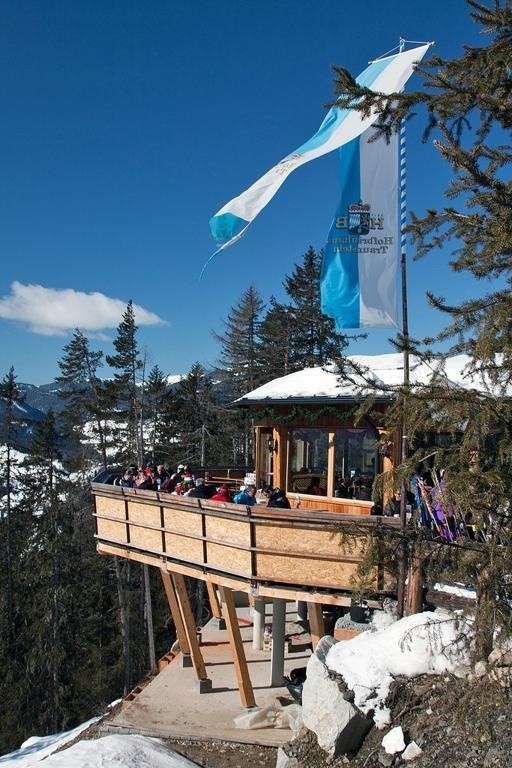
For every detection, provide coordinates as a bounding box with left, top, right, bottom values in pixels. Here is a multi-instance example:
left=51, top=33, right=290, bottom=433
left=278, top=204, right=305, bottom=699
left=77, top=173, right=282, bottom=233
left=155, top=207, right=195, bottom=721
left=232, top=485, right=257, bottom=506
left=335, top=468, right=401, bottom=516
left=210, top=485, right=232, bottom=502
left=118, top=462, right=196, bottom=496
left=262, top=484, right=290, bottom=508
left=188, top=477, right=213, bottom=499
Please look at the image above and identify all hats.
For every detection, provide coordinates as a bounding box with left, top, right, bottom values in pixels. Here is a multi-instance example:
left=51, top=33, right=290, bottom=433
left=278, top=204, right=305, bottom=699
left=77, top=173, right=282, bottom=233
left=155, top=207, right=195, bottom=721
left=219, top=486, right=229, bottom=492
left=184, top=477, right=193, bottom=484
